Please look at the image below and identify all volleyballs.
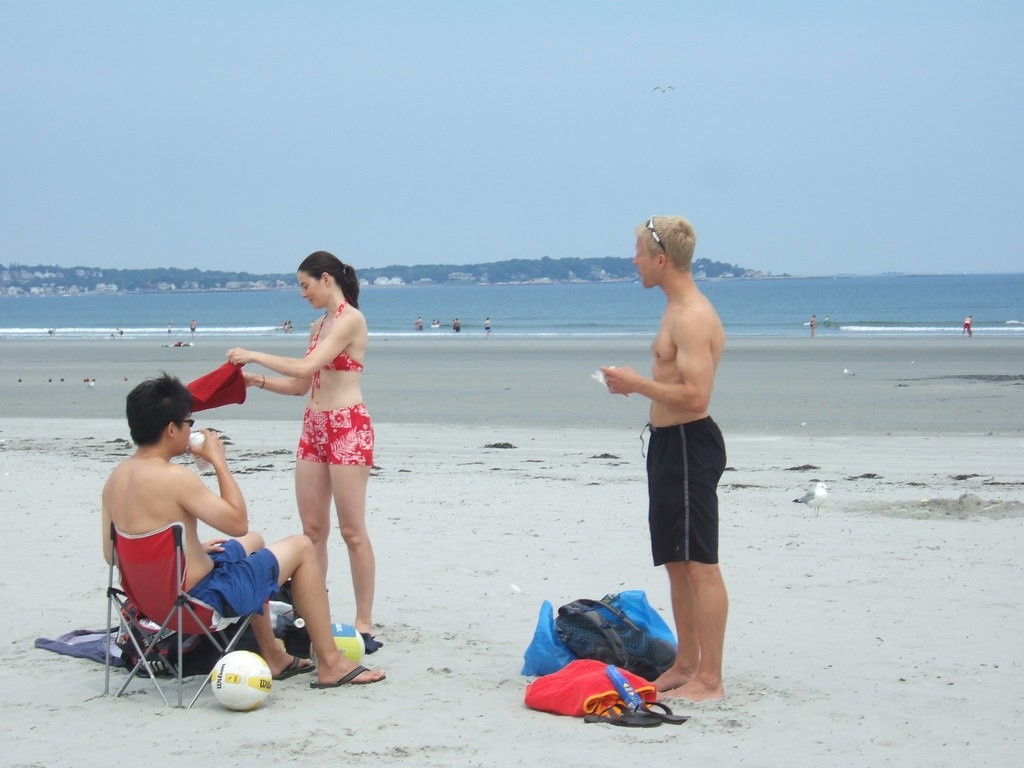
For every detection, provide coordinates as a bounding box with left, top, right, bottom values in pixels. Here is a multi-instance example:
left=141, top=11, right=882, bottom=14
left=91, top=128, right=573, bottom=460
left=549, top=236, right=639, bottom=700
left=211, top=650, right=272, bottom=711
left=310, top=623, right=365, bottom=666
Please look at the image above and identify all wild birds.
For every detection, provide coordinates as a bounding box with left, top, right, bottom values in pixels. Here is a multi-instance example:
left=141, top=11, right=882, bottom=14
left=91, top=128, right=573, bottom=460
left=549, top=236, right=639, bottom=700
left=792, top=482, right=831, bottom=518
left=843, top=369, right=855, bottom=377
left=87, top=381, right=95, bottom=389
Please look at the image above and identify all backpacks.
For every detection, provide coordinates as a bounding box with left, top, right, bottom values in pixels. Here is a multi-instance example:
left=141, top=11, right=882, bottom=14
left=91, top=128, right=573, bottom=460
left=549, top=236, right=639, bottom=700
left=521, top=590, right=676, bottom=679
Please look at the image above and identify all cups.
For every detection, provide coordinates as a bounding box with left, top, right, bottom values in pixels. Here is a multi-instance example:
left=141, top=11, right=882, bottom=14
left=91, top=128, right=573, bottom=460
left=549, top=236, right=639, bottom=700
left=188, top=432, right=212, bottom=471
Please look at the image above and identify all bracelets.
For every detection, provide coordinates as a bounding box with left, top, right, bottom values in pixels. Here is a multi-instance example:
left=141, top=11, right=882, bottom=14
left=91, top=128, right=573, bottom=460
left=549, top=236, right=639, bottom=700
left=259, top=375, right=266, bottom=388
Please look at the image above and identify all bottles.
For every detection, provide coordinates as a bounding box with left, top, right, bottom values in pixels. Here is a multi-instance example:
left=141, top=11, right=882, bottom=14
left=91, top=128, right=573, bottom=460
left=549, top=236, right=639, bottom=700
left=604, top=664, right=644, bottom=711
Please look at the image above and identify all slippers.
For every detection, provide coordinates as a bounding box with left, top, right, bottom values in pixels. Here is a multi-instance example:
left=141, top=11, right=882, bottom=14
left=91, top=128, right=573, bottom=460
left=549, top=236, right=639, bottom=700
left=273, top=655, right=318, bottom=680
left=583, top=702, right=662, bottom=726
left=643, top=701, right=691, bottom=724
left=310, top=665, right=388, bottom=687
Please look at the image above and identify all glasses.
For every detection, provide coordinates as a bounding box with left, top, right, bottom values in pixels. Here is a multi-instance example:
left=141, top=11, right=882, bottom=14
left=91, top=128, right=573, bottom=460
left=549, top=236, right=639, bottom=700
left=172, top=417, right=195, bottom=428
left=646, top=215, right=668, bottom=255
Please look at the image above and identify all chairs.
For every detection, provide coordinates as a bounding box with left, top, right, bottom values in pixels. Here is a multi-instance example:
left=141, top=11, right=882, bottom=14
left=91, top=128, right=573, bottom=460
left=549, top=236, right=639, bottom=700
left=104, top=520, right=269, bottom=709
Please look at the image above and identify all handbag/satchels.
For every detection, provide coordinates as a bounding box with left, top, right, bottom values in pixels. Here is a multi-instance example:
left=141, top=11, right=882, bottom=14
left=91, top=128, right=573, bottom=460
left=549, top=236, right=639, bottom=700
left=114, top=598, right=227, bottom=678
left=527, top=659, right=658, bottom=717
left=276, top=608, right=313, bottom=653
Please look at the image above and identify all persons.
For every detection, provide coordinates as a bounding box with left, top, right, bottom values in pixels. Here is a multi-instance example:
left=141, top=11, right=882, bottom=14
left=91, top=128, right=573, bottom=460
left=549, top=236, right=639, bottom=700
left=823, top=315, right=830, bottom=327
left=168, top=321, right=172, bottom=334
left=282, top=320, right=292, bottom=332
left=484, top=318, right=491, bottom=338
left=962, top=316, right=972, bottom=335
left=433, top=320, right=439, bottom=325
left=811, top=315, right=816, bottom=336
left=226, top=251, right=376, bottom=637
left=102, top=372, right=385, bottom=688
left=191, top=320, right=196, bottom=336
left=415, top=318, right=423, bottom=331
left=453, top=318, right=460, bottom=332
left=600, top=216, right=728, bottom=701
left=111, top=328, right=123, bottom=340
